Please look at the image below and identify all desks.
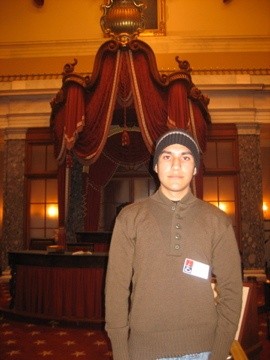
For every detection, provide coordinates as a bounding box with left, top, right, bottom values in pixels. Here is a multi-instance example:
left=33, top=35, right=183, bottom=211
left=0, top=250, right=109, bottom=328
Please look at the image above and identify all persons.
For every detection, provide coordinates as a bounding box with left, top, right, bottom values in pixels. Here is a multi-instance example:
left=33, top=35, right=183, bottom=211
left=104, top=131, right=244, bottom=360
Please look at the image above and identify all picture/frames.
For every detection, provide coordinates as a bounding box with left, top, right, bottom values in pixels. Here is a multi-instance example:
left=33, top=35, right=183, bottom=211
left=136, top=0, right=166, bottom=37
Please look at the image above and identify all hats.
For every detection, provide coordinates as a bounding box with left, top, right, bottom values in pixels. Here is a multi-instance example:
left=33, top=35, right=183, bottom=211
left=153, top=128, right=201, bottom=171
